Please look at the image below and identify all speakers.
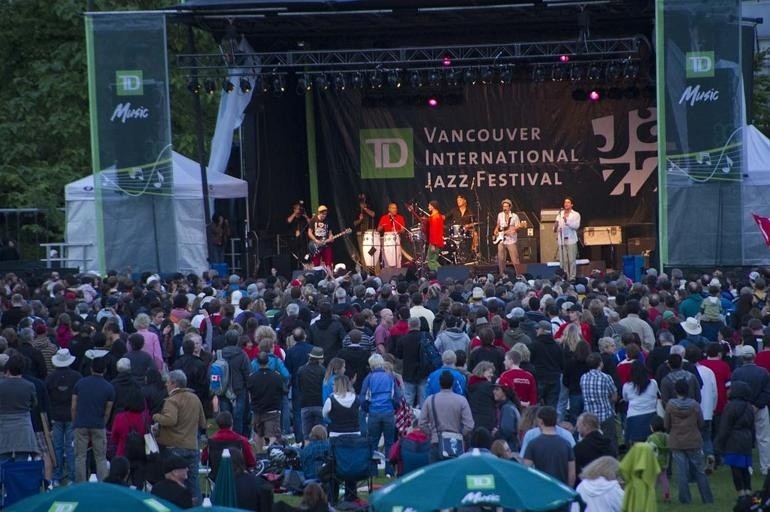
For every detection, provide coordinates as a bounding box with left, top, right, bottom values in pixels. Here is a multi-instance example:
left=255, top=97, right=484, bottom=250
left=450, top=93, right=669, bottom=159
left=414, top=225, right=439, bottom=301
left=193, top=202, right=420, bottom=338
left=526, top=263, right=560, bottom=279
left=292, top=270, right=316, bottom=281
left=622, top=255, right=642, bottom=283
left=436, top=266, right=471, bottom=281
left=379, top=268, right=408, bottom=283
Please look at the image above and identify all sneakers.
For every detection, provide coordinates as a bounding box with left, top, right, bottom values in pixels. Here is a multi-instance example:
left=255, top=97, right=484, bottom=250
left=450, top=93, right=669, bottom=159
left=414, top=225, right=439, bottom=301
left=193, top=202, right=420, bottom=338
left=734, top=496, right=747, bottom=512
left=749, top=495, right=753, bottom=505
left=704, top=455, right=715, bottom=475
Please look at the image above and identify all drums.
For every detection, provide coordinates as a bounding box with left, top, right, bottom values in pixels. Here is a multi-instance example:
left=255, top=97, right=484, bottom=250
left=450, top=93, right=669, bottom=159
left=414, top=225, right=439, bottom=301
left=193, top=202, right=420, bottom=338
left=362, top=229, right=381, bottom=267
left=438, top=237, right=462, bottom=265
left=448, top=224, right=471, bottom=241
left=382, top=231, right=401, bottom=269
left=409, top=226, right=424, bottom=242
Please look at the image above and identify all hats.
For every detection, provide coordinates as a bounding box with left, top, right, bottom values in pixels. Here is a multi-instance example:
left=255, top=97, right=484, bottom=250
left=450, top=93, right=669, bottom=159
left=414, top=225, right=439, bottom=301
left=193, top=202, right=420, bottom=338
left=106, top=296, right=118, bottom=306
left=366, top=287, right=376, bottom=295
left=308, top=346, right=324, bottom=358
left=230, top=291, right=242, bottom=304
left=334, top=263, right=346, bottom=273
left=567, top=303, right=582, bottom=312
left=165, top=455, right=193, bottom=471
left=473, top=286, right=483, bottom=299
left=534, top=321, right=552, bottom=330
left=570, top=277, right=588, bottom=285
left=318, top=206, right=328, bottom=212
left=647, top=268, right=762, bottom=356
left=291, top=280, right=301, bottom=286
left=505, top=307, right=525, bottom=318
left=501, top=199, right=513, bottom=210
left=52, top=348, right=77, bottom=368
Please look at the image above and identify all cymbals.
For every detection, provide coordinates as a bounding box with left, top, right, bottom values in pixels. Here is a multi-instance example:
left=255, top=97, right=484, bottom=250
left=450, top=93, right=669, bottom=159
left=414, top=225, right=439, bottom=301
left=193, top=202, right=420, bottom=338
left=466, top=221, right=485, bottom=228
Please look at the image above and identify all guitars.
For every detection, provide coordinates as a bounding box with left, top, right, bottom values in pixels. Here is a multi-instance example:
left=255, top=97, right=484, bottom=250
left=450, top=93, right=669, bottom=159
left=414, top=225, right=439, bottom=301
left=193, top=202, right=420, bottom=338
left=492, top=220, right=527, bottom=246
left=308, top=227, right=352, bottom=256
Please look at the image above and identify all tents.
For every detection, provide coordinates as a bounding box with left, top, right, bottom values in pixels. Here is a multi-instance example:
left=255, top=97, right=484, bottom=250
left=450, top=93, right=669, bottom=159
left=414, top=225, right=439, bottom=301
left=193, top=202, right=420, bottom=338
left=65, top=149, right=249, bottom=277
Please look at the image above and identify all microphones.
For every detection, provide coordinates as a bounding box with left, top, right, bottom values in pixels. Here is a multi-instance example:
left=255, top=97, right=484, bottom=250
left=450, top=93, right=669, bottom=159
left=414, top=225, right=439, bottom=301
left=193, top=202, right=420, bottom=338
left=504, top=209, right=507, bottom=213
left=415, top=203, right=417, bottom=212
left=389, top=213, right=393, bottom=227
left=559, top=207, right=563, bottom=215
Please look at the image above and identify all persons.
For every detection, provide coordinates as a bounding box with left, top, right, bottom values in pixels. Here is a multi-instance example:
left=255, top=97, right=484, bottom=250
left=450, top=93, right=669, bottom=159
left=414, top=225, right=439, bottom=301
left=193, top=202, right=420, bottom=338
left=237, top=212, right=258, bottom=250
left=352, top=199, right=376, bottom=260
left=288, top=202, right=310, bottom=268
left=443, top=194, right=475, bottom=229
left=207, top=212, right=230, bottom=265
left=553, top=196, right=581, bottom=281
left=308, top=205, right=334, bottom=267
left=376, top=203, right=406, bottom=269
left=0, top=263, right=769, bottom=512
left=409, top=200, right=445, bottom=272
left=494, top=199, right=522, bottom=276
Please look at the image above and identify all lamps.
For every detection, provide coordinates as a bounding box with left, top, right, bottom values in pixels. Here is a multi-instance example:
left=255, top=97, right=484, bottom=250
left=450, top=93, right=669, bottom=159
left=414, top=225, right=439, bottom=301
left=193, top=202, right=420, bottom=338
left=171, top=51, right=651, bottom=96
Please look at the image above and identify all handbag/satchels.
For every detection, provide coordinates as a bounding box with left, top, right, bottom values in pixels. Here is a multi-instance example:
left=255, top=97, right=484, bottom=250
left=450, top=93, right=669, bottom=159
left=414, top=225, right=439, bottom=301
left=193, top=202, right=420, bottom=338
left=437, top=430, right=464, bottom=459
left=274, top=370, right=289, bottom=393
left=144, top=432, right=160, bottom=460
left=508, top=436, right=522, bottom=451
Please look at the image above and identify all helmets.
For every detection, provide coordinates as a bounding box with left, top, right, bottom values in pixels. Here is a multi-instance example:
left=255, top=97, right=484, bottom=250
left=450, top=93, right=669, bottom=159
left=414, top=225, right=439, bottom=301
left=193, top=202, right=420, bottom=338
left=490, top=377, right=515, bottom=390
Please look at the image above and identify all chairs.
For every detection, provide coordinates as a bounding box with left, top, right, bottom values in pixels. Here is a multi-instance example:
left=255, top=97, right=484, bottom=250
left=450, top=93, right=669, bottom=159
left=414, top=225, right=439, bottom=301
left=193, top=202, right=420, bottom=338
left=205, top=436, right=244, bottom=495
left=0, top=459, right=46, bottom=510
left=394, top=436, right=431, bottom=479
left=328, top=433, right=374, bottom=505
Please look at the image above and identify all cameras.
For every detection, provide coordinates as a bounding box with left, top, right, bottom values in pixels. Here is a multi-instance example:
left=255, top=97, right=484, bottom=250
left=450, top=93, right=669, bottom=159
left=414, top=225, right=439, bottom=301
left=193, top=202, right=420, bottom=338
left=299, top=200, right=304, bottom=214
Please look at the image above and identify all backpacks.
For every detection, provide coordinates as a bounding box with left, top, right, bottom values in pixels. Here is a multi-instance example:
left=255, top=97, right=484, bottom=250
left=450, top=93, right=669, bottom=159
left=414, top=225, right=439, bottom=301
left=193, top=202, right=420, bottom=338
left=125, top=414, right=145, bottom=461
left=753, top=293, right=768, bottom=308
left=414, top=332, right=442, bottom=382
left=207, top=349, right=237, bottom=400
